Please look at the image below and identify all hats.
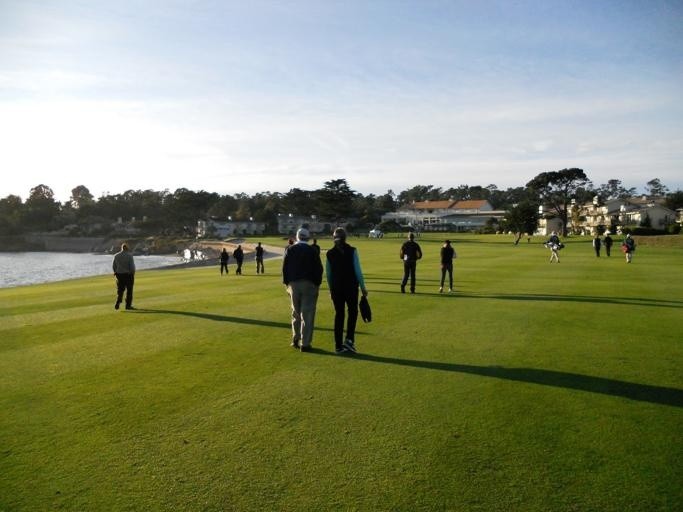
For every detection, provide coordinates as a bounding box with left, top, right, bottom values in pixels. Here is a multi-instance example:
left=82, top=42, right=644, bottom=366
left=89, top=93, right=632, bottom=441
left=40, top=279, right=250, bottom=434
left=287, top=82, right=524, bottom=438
left=295, top=228, right=310, bottom=239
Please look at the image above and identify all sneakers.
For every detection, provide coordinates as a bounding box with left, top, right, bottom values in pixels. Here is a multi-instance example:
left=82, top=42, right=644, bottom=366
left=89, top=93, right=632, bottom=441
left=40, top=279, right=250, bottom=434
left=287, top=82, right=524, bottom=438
left=401, top=286, right=452, bottom=293
left=342, top=340, right=357, bottom=352
left=334, top=347, right=347, bottom=354
left=289, top=342, right=299, bottom=349
left=299, top=345, right=311, bottom=352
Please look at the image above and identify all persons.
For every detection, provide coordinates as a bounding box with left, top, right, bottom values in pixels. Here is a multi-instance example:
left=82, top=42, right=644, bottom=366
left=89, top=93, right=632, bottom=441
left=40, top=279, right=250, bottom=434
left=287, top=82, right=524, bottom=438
left=282, top=228, right=324, bottom=351
left=219, top=248, right=229, bottom=274
left=284, top=238, right=294, bottom=251
left=326, top=228, right=369, bottom=353
left=233, top=245, right=244, bottom=274
left=256, top=242, right=265, bottom=274
left=112, top=243, right=136, bottom=310
left=439, top=240, right=457, bottom=292
left=543, top=231, right=561, bottom=263
left=603, top=233, right=613, bottom=256
left=400, top=232, right=422, bottom=293
left=312, top=238, right=319, bottom=247
left=621, top=234, right=635, bottom=262
left=593, top=233, right=602, bottom=257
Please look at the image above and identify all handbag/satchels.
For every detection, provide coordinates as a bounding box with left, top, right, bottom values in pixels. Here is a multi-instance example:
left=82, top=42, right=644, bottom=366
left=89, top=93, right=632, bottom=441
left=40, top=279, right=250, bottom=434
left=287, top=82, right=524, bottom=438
left=544, top=241, right=565, bottom=251
left=359, top=295, right=371, bottom=322
